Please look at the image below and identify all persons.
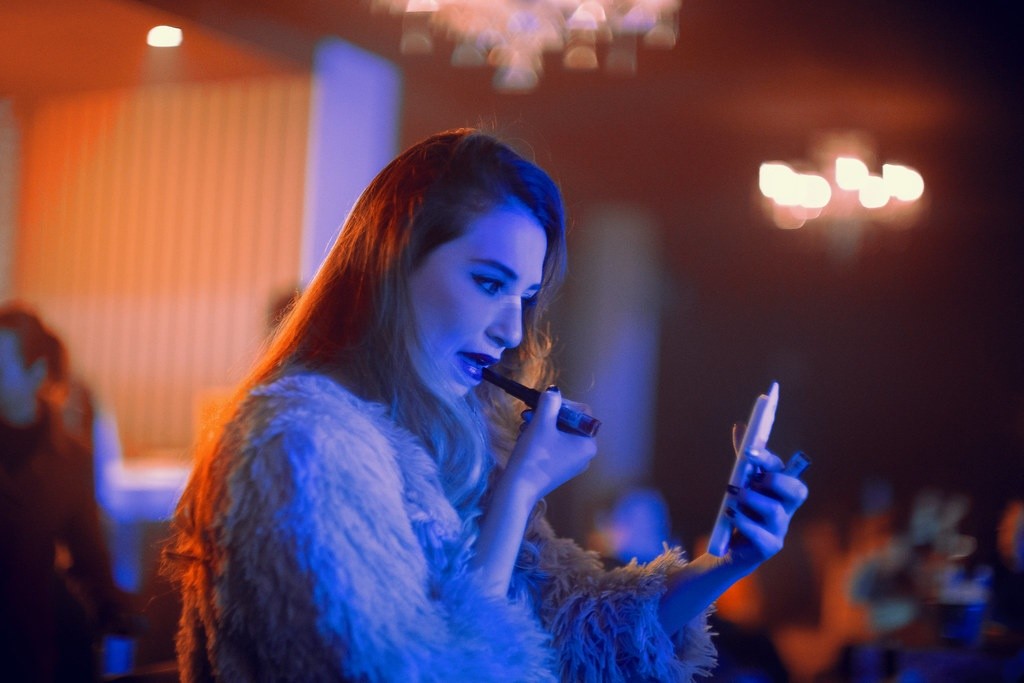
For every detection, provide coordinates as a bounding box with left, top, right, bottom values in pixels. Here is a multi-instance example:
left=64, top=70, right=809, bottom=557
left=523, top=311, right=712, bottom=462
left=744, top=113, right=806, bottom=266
left=160, top=130, right=808, bottom=683
left=572, top=460, right=1024, bottom=683
left=0, top=309, right=144, bottom=683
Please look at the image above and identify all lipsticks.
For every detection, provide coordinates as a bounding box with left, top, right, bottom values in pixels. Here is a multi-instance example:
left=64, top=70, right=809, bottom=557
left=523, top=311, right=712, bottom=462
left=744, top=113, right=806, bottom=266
left=481, top=367, right=601, bottom=437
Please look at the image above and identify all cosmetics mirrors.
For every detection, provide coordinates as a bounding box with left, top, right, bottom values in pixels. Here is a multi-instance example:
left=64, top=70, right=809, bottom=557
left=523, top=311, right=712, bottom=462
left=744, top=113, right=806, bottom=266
left=707, top=383, right=779, bottom=556
